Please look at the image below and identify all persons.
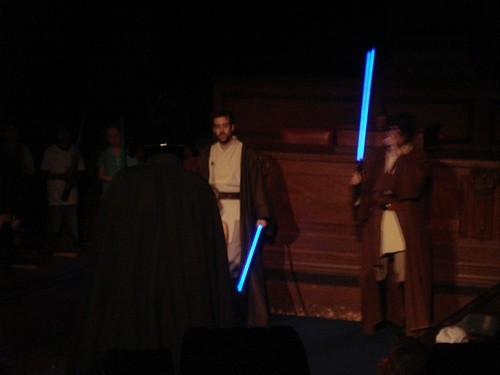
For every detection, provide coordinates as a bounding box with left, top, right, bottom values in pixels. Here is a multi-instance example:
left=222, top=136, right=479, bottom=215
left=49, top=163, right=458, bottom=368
left=0, top=125, right=129, bottom=243
left=198, top=112, right=273, bottom=326
left=363, top=112, right=433, bottom=336
